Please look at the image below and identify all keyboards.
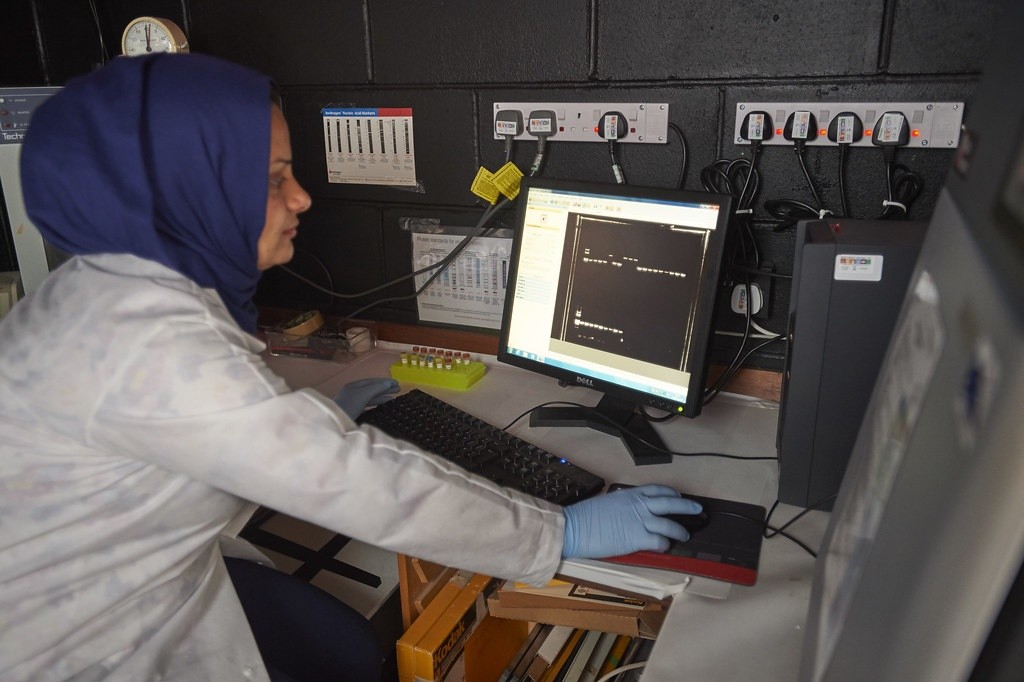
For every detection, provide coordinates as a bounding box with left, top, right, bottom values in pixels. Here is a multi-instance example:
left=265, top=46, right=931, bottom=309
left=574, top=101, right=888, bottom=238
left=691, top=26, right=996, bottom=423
left=354, top=388, right=606, bottom=508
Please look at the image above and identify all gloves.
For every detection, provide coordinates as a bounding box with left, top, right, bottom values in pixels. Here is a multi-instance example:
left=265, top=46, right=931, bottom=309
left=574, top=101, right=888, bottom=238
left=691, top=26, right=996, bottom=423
left=558, top=483, right=703, bottom=560
left=334, top=377, right=401, bottom=420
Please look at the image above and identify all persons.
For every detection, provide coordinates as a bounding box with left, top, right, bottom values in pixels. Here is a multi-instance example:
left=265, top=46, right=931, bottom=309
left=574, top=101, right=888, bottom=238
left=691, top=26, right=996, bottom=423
left=0, top=52, right=703, bottom=682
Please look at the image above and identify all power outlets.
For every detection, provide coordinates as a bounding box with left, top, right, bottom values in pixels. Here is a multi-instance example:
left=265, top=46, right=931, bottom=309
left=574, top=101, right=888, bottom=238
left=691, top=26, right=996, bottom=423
left=492, top=101, right=669, bottom=145
left=713, top=267, right=771, bottom=318
left=733, top=101, right=963, bottom=148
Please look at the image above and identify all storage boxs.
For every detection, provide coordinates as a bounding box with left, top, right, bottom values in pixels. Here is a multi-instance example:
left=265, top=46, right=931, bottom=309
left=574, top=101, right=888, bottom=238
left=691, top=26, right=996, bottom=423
left=394, top=567, right=675, bottom=682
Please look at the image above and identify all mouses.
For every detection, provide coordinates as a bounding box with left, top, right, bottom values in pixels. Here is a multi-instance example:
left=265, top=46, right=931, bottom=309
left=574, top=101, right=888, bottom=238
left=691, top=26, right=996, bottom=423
left=663, top=510, right=709, bottom=531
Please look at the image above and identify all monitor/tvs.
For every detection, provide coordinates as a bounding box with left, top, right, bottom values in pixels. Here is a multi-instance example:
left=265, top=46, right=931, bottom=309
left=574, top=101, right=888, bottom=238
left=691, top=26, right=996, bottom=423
left=497, top=176, right=734, bottom=466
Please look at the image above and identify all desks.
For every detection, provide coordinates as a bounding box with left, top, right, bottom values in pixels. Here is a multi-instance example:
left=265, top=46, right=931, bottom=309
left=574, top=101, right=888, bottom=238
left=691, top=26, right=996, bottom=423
left=255, top=331, right=832, bottom=682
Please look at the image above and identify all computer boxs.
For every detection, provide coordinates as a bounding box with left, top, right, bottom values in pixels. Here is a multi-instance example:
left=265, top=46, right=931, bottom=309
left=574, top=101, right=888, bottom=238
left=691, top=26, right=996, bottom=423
left=777, top=217, right=928, bottom=513
